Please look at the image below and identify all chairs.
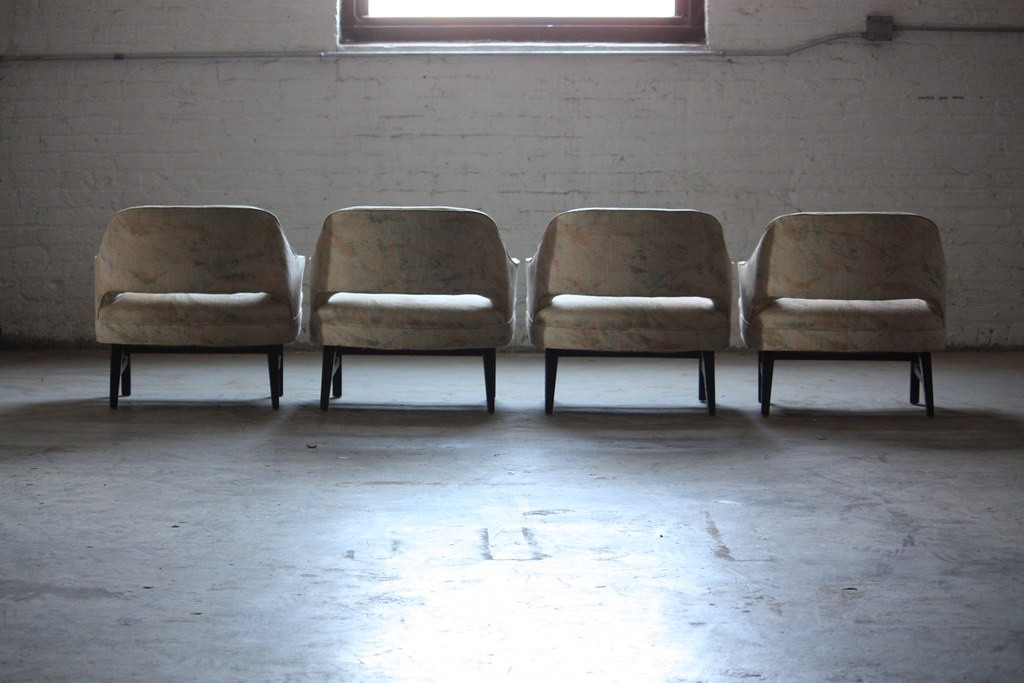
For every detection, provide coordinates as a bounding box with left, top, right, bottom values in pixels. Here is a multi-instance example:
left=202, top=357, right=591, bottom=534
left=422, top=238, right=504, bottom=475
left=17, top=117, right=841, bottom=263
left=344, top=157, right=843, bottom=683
left=93, top=206, right=305, bottom=411
left=525, top=208, right=734, bottom=417
left=738, top=212, right=946, bottom=420
left=309, top=205, right=520, bottom=413
